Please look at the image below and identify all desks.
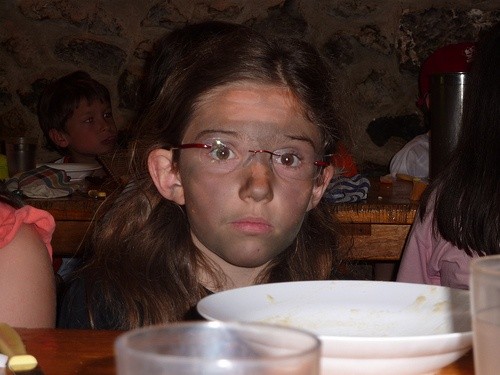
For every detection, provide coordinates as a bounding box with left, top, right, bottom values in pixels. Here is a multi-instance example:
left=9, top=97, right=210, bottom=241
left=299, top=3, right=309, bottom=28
left=25, top=183, right=421, bottom=261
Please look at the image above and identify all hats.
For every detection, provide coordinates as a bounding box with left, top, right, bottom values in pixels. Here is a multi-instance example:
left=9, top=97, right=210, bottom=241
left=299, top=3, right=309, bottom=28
left=416, top=41, right=480, bottom=106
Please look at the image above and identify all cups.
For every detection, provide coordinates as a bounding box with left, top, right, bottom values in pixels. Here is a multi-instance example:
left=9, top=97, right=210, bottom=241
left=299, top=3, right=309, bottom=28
left=114, top=320, right=321, bottom=375
left=469, top=254, right=500, bottom=375
left=4, top=135, right=39, bottom=179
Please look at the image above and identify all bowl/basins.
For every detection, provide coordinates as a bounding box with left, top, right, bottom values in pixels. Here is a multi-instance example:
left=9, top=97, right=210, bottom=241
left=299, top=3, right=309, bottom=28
left=197, top=279, right=473, bottom=375
left=36, top=162, right=104, bottom=181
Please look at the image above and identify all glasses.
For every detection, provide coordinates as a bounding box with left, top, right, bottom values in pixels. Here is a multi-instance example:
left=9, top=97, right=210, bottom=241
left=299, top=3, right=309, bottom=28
left=173, top=141, right=330, bottom=181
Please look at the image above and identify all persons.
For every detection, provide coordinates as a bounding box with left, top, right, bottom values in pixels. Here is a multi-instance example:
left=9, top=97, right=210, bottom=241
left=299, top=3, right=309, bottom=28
left=63, top=30, right=340, bottom=334
left=383, top=41, right=475, bottom=179
left=394, top=29, right=500, bottom=292
left=36, top=73, right=120, bottom=272
left=0, top=202, right=56, bottom=328
left=118, top=20, right=372, bottom=284
